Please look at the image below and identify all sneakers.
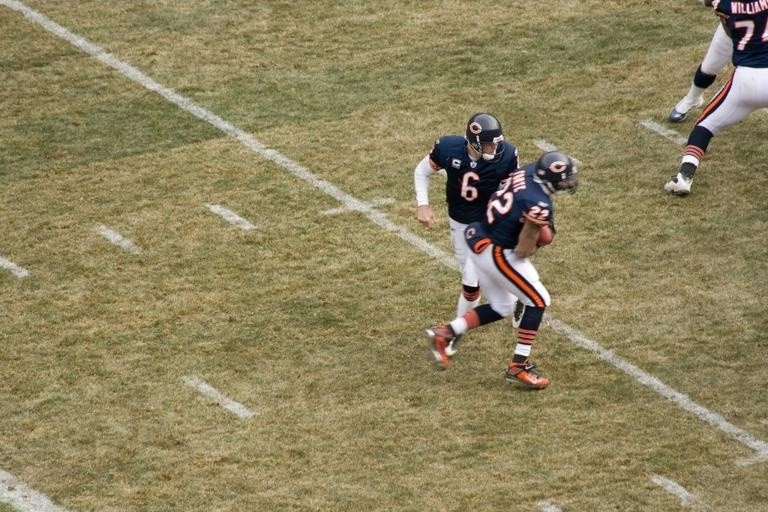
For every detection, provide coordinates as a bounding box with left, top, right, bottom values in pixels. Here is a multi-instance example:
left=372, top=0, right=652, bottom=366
left=423, top=321, right=456, bottom=371
left=669, top=94, right=705, bottom=122
left=662, top=172, right=694, bottom=196
left=511, top=299, right=524, bottom=329
left=502, top=365, right=551, bottom=390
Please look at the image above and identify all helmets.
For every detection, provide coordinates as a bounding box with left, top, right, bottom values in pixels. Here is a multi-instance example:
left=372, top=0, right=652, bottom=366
left=532, top=151, right=579, bottom=198
left=466, top=112, right=506, bottom=162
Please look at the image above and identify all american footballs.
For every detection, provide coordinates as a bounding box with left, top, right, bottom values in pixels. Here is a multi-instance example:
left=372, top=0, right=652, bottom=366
left=536, top=227, right=553, bottom=246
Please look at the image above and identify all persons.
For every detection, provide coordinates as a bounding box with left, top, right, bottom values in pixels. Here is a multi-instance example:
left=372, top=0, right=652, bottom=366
left=414, top=112, right=519, bottom=356
left=663, top=0, right=768, bottom=195
left=425, top=151, right=577, bottom=389
left=669, top=21, right=734, bottom=121
